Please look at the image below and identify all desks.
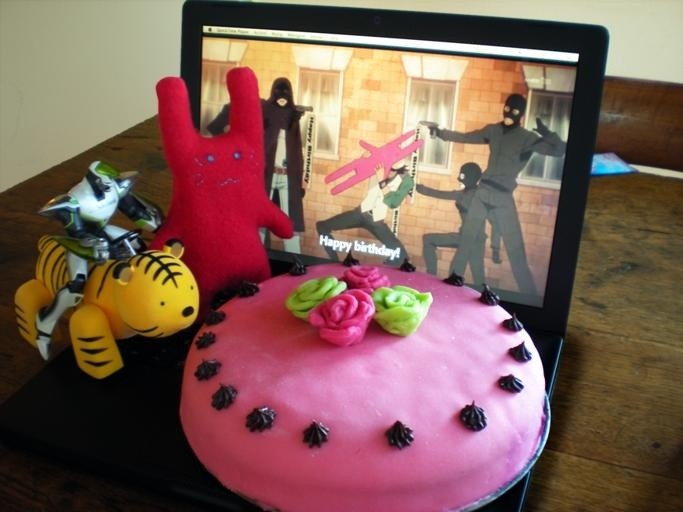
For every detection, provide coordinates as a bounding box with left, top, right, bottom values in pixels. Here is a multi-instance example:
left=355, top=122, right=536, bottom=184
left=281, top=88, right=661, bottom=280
left=0, top=75, right=683, bottom=512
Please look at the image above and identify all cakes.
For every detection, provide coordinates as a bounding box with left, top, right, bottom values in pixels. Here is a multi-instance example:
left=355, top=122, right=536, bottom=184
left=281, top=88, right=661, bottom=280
left=177, top=250, right=547, bottom=512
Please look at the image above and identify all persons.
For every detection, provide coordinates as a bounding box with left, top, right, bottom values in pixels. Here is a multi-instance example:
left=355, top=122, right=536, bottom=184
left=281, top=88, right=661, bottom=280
left=34, top=160, right=166, bottom=361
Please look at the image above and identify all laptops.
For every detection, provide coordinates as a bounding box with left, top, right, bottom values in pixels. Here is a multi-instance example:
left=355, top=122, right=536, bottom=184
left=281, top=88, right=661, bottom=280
left=0, top=0, right=609, bottom=512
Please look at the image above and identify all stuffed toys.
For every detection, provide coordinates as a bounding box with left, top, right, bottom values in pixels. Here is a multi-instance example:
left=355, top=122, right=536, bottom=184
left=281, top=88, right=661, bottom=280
left=151, top=65, right=294, bottom=322
